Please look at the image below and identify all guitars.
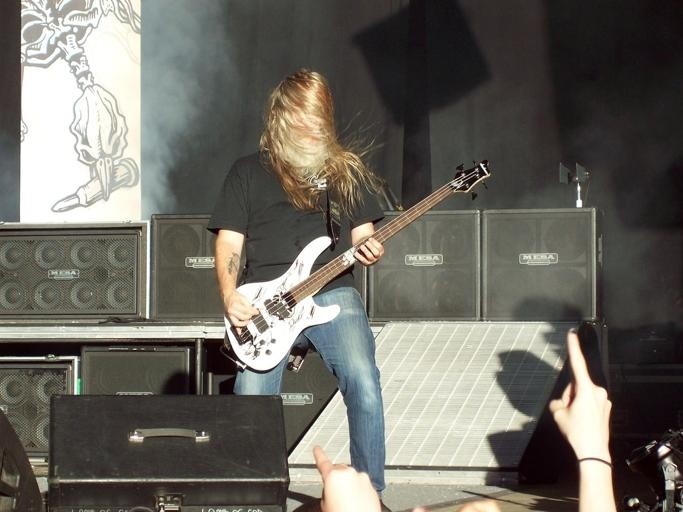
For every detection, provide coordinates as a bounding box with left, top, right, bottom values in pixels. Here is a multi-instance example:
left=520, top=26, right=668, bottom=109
left=224, top=160, right=489, bottom=373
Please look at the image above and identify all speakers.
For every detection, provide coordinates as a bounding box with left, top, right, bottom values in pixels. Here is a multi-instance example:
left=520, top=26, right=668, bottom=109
left=212, top=349, right=339, bottom=457
left=0, top=354, right=81, bottom=457
left=81, top=345, right=195, bottom=396
left=367, top=209, right=481, bottom=321
left=0, top=409, right=45, bottom=512
left=150, top=213, right=227, bottom=322
left=0, top=220, right=150, bottom=327
left=481, top=207, right=597, bottom=321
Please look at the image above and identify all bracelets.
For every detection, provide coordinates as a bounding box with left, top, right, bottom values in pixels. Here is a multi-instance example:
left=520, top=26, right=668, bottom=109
left=575, top=458, right=614, bottom=472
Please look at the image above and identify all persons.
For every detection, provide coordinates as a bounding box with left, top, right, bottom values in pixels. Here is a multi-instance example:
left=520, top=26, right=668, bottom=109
left=313, top=446, right=381, bottom=511
left=204, top=67, right=392, bottom=512
left=550, top=328, right=616, bottom=511
